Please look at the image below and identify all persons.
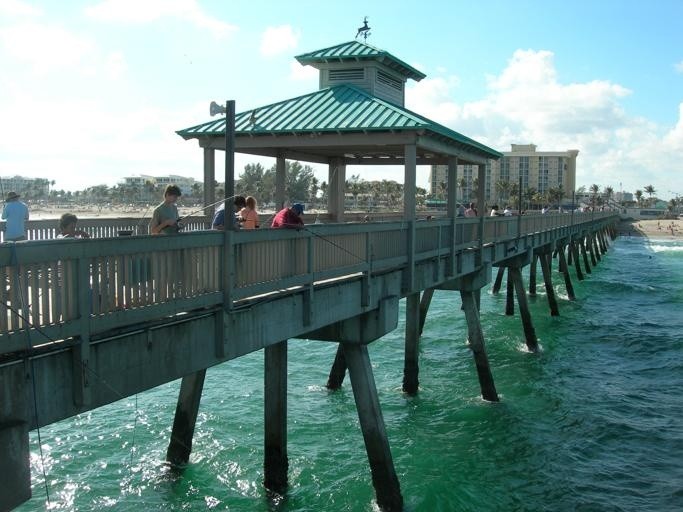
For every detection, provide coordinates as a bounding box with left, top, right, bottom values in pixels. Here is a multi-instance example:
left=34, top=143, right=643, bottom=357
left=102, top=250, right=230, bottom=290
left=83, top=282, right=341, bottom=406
left=239, top=193, right=260, bottom=230
left=541, top=205, right=550, bottom=215
left=270, top=202, right=306, bottom=230
left=0, top=192, right=29, bottom=280
left=502, top=205, right=513, bottom=217
left=53, top=213, right=90, bottom=322
left=211, top=197, right=245, bottom=230
left=488, top=205, right=505, bottom=216
left=461, top=202, right=477, bottom=217
left=147, top=184, right=187, bottom=302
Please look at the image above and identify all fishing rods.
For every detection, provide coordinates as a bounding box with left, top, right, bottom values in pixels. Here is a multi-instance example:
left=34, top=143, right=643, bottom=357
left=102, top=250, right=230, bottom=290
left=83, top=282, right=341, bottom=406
left=0, top=301, right=112, bottom=383
left=305, top=228, right=371, bottom=262
left=315, top=168, right=338, bottom=225
left=0, top=177, right=5, bottom=208
left=178, top=194, right=241, bottom=221
left=243, top=219, right=304, bottom=226
left=456, top=200, right=467, bottom=210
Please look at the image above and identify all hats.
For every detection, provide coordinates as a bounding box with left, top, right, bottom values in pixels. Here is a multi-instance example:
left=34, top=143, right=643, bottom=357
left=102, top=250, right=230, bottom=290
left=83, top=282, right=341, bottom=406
left=5, top=191, right=19, bottom=201
left=293, top=203, right=305, bottom=215
